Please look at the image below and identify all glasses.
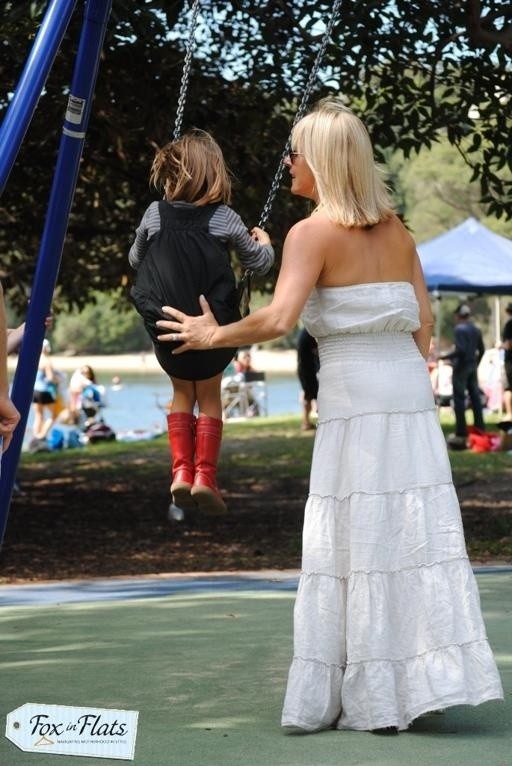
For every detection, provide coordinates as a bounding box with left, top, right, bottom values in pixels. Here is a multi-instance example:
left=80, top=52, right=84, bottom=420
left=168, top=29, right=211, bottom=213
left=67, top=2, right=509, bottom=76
left=288, top=148, right=304, bottom=164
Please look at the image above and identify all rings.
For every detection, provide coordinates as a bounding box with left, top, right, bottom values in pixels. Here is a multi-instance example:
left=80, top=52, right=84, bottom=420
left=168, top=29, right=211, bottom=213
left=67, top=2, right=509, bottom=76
left=171, top=333, right=177, bottom=342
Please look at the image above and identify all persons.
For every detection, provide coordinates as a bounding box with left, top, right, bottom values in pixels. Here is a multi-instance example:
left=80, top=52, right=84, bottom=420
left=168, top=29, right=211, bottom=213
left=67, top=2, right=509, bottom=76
left=292, top=321, right=323, bottom=432
left=491, top=300, right=512, bottom=430
left=124, top=123, right=277, bottom=519
left=5, top=307, right=54, bottom=357
left=0, top=282, right=54, bottom=460
left=154, top=93, right=511, bottom=733
left=434, top=301, right=490, bottom=448
left=31, top=338, right=122, bottom=451
left=231, top=349, right=263, bottom=418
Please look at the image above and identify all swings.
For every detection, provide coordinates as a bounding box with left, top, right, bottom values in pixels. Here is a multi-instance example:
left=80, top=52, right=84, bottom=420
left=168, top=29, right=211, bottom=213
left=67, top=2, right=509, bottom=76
left=134, top=2, right=346, bottom=382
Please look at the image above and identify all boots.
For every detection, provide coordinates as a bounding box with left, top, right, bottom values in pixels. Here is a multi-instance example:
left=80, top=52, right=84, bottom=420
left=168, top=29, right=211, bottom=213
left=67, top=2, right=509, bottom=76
left=191, top=415, right=227, bottom=515
left=166, top=411, right=197, bottom=510
